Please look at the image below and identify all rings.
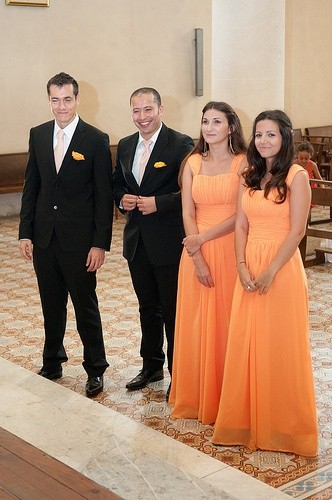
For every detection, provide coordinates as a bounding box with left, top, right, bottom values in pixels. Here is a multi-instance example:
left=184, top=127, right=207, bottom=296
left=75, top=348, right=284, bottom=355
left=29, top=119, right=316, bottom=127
left=247, top=285, right=251, bottom=289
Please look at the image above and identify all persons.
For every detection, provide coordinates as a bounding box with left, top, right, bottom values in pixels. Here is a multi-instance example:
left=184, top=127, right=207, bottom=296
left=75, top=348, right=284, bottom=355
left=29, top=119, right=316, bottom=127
left=16, top=71, right=113, bottom=396
left=167, top=101, right=249, bottom=424
left=293, top=142, right=324, bottom=208
left=112, top=87, right=196, bottom=401
left=212, top=110, right=319, bottom=458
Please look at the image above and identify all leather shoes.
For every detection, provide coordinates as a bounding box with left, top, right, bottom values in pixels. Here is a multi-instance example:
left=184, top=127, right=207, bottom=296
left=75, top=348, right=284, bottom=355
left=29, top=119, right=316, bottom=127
left=86, top=376, right=103, bottom=397
left=126, top=368, right=164, bottom=390
left=38, top=363, right=62, bottom=380
left=166, top=382, right=173, bottom=401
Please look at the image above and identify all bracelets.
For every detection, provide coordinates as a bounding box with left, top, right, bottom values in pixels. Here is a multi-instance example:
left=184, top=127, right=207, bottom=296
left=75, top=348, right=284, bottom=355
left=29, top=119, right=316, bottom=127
left=236, top=261, right=246, bottom=267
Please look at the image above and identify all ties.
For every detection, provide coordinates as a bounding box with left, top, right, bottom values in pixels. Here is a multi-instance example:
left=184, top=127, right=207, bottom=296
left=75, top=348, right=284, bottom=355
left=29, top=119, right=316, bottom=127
left=54, top=130, right=67, bottom=174
left=139, top=141, right=152, bottom=185
